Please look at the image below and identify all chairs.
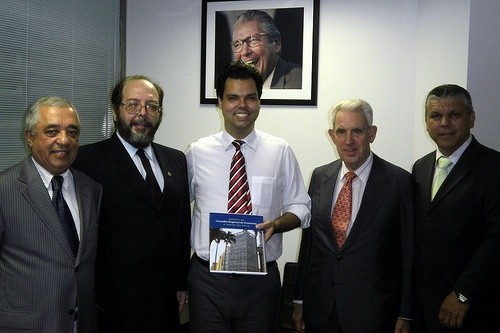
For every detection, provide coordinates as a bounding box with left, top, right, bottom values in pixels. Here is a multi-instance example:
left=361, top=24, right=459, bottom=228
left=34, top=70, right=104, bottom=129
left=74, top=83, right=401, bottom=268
left=275, top=262, right=299, bottom=333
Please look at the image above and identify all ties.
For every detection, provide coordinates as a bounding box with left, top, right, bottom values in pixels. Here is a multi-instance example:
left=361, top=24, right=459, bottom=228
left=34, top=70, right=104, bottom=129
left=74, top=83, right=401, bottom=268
left=227, top=140, right=253, bottom=215
left=51, top=175, right=79, bottom=254
left=137, top=148, right=161, bottom=193
left=331, top=172, right=357, bottom=248
left=431, top=157, right=453, bottom=200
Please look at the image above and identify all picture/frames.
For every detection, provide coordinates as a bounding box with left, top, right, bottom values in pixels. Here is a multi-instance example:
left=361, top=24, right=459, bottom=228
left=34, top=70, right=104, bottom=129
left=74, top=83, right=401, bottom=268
left=199, top=0, right=320, bottom=107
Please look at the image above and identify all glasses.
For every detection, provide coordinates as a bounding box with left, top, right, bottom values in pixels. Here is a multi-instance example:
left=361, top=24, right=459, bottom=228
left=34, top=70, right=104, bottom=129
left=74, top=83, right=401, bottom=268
left=231, top=33, right=262, bottom=54
left=123, top=100, right=162, bottom=116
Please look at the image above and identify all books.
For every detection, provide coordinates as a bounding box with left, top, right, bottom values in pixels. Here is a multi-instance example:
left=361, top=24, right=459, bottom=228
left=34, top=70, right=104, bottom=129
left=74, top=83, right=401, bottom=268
left=209, top=212, right=267, bottom=275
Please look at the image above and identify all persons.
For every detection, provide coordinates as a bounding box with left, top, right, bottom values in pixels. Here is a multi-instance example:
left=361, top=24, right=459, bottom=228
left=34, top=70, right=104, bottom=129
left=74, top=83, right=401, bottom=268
left=182, top=61, right=311, bottom=332
left=413, top=86, right=500, bottom=333
left=296, top=99, right=417, bottom=333
left=230, top=10, right=302, bottom=89
left=0, top=96, right=102, bottom=333
left=68, top=74, right=192, bottom=333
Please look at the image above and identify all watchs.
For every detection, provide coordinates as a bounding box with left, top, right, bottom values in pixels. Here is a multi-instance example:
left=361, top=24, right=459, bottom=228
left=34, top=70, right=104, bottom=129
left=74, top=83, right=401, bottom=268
left=455, top=290, right=470, bottom=304
